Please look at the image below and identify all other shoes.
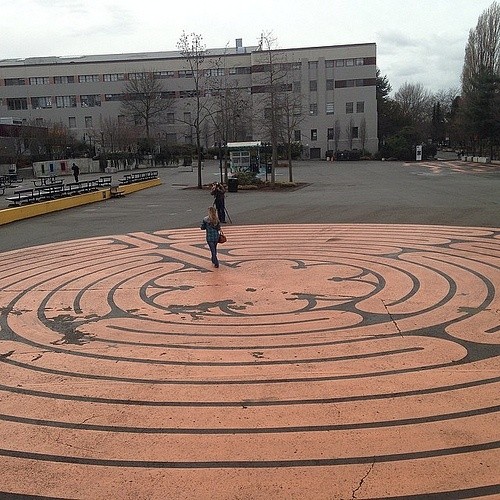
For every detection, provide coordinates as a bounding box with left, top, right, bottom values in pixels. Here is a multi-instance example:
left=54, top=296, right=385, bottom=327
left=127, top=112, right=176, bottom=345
left=215, top=264, right=219, bottom=268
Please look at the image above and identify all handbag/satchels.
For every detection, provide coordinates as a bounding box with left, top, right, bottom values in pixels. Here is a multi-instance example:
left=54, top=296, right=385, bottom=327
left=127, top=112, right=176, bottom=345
left=218, top=230, right=226, bottom=243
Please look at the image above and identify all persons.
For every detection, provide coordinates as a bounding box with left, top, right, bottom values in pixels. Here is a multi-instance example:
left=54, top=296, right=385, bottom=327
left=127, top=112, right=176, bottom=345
left=211, top=181, right=227, bottom=223
left=201, top=207, right=223, bottom=268
left=72, top=163, right=80, bottom=182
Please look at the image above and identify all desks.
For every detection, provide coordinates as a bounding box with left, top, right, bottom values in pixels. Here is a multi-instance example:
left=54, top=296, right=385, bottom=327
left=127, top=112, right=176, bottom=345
left=4, top=173, right=16, bottom=185
left=37, top=175, right=50, bottom=184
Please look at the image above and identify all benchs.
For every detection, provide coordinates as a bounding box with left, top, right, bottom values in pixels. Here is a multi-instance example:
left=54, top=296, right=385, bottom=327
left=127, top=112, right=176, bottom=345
left=1, top=170, right=158, bottom=206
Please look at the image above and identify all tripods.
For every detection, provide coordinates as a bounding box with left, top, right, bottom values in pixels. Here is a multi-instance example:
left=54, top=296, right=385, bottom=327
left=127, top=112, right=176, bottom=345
left=213, top=190, right=232, bottom=224
